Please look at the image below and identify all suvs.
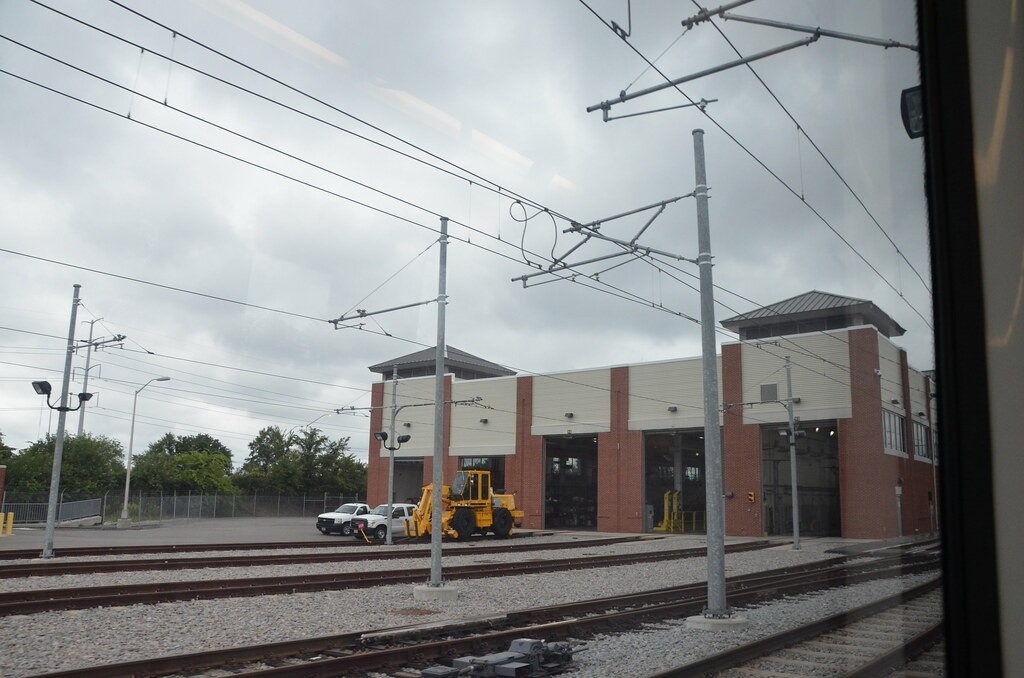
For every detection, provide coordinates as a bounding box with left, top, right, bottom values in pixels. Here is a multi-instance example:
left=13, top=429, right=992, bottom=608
left=350, top=503, right=419, bottom=539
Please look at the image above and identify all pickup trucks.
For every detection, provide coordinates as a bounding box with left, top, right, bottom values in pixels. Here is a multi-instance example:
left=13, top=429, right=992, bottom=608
left=316, top=503, right=374, bottom=536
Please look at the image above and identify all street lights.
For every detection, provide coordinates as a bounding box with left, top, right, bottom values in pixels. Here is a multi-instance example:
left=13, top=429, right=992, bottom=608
left=116, top=376, right=170, bottom=530
left=373, top=364, right=411, bottom=544
left=32, top=285, right=94, bottom=558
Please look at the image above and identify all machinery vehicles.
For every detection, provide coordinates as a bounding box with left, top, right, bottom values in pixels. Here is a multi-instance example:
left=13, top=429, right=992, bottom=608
left=403, top=471, right=525, bottom=541
